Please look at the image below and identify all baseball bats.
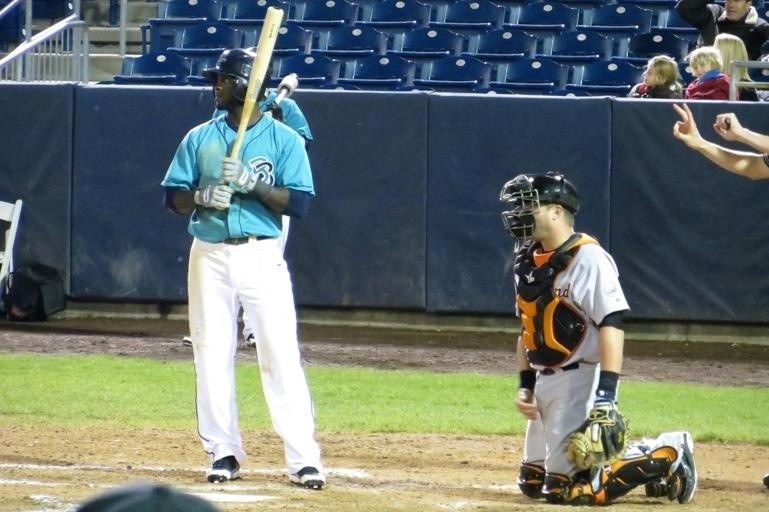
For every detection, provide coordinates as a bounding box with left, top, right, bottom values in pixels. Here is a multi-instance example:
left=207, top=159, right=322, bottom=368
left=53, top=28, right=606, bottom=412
left=214, top=5, right=284, bottom=212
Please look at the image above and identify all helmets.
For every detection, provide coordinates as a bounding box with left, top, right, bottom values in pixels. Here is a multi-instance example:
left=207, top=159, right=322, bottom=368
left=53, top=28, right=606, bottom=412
left=200, top=46, right=266, bottom=103
left=504, top=173, right=581, bottom=240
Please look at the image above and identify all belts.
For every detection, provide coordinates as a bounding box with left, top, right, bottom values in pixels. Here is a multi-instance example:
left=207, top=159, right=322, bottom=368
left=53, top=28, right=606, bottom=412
left=219, top=234, right=269, bottom=245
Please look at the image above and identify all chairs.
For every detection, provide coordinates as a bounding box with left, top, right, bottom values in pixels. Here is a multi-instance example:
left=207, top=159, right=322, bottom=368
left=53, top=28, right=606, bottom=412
left=113, top=1, right=705, bottom=99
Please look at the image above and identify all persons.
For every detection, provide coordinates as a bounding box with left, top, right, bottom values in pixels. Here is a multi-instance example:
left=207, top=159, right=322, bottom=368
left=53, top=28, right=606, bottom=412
left=674, top=0, right=769, bottom=84
left=629, top=54, right=681, bottom=103
left=159, top=48, right=330, bottom=492
left=668, top=98, right=768, bottom=182
left=708, top=33, right=758, bottom=103
left=498, top=168, right=705, bottom=508
left=210, top=81, right=314, bottom=349
left=680, top=47, right=740, bottom=104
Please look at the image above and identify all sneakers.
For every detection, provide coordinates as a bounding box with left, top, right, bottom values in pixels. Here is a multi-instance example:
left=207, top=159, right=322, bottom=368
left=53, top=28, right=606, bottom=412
left=288, top=468, right=325, bottom=489
left=182, top=334, right=192, bottom=347
left=246, top=335, right=256, bottom=347
left=671, top=429, right=696, bottom=505
left=642, top=432, right=669, bottom=497
left=207, top=455, right=241, bottom=481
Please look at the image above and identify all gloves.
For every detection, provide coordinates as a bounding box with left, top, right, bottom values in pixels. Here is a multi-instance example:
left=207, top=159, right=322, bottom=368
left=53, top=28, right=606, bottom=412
left=220, top=158, right=257, bottom=193
left=194, top=184, right=233, bottom=210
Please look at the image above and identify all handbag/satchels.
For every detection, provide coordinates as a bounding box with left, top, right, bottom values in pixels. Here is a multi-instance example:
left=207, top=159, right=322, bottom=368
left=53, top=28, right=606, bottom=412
left=6, top=261, right=66, bottom=322
